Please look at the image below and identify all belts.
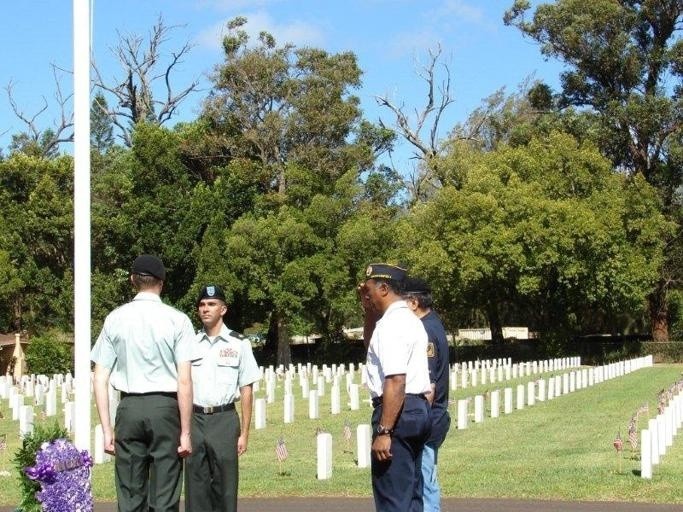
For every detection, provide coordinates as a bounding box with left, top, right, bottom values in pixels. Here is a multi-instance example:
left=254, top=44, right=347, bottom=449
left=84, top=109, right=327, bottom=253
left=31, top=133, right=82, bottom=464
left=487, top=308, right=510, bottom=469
left=371, top=397, right=383, bottom=408
left=195, top=403, right=235, bottom=414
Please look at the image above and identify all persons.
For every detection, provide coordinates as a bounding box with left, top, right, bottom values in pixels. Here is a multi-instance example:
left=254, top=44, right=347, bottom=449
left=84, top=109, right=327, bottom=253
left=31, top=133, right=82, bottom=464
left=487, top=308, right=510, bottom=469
left=182, top=286, right=261, bottom=512
left=358, top=263, right=450, bottom=511
left=91, top=254, right=202, bottom=512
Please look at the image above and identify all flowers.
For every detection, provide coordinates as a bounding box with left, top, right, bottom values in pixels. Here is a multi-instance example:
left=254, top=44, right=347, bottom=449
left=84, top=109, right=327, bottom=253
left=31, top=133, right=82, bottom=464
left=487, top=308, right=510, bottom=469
left=27, top=436, right=94, bottom=512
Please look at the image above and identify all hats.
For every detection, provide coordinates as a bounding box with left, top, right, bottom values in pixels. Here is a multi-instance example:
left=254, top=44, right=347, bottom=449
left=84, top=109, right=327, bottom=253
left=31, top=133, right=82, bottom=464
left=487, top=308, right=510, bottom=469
left=365, top=263, right=427, bottom=295
left=198, top=285, right=226, bottom=303
left=131, top=255, right=166, bottom=281
